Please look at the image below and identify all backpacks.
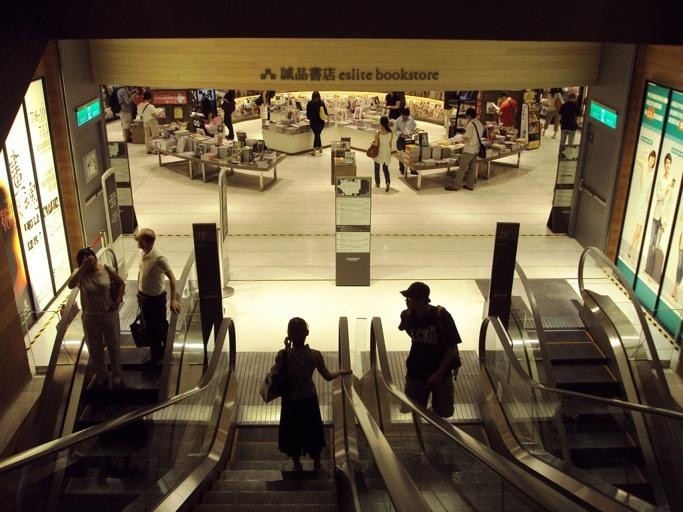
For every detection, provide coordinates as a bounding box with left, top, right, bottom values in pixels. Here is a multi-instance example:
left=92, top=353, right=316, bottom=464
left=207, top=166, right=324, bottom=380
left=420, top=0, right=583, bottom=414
left=405, top=306, right=461, bottom=380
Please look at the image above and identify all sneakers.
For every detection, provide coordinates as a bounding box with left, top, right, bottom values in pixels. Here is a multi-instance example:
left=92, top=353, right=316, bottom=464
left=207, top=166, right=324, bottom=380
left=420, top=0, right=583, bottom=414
left=111, top=376, right=121, bottom=385
left=444, top=186, right=459, bottom=191
left=96, top=374, right=104, bottom=385
left=463, top=185, right=473, bottom=190
left=385, top=182, right=389, bottom=192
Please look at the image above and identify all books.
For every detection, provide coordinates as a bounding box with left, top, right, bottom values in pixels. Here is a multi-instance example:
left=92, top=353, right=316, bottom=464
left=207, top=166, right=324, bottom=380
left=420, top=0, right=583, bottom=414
left=399, top=122, right=528, bottom=166
left=151, top=125, right=277, bottom=168
left=523, top=92, right=540, bottom=134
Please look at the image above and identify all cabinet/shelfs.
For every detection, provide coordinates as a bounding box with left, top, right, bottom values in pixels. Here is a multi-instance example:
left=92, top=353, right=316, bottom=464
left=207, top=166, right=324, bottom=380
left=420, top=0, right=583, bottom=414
left=520, top=100, right=542, bottom=151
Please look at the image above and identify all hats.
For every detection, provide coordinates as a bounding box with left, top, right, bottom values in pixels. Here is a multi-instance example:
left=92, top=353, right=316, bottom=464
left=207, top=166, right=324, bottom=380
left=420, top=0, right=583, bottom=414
left=400, top=282, right=430, bottom=301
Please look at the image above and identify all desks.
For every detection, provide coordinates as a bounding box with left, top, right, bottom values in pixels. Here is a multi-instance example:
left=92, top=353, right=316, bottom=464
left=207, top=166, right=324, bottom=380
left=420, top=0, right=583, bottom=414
left=394, top=132, right=526, bottom=190
left=152, top=133, right=287, bottom=192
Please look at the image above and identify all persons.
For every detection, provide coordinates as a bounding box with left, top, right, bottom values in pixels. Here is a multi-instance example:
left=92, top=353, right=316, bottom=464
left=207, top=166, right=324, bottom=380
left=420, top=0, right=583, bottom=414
left=67, top=246, right=125, bottom=386
left=254, top=90, right=275, bottom=126
left=106, top=85, right=137, bottom=141
left=541, top=88, right=564, bottom=139
left=214, top=89, right=236, bottom=140
left=381, top=91, right=406, bottom=119
left=643, top=152, right=675, bottom=276
left=366, top=115, right=393, bottom=192
left=558, top=92, right=579, bottom=153
left=258, top=317, right=352, bottom=469
left=670, top=232, right=683, bottom=300
left=127, top=228, right=181, bottom=364
left=490, top=92, right=518, bottom=128
left=394, top=107, right=418, bottom=175
left=442, top=107, right=486, bottom=191
left=396, top=281, right=463, bottom=425
left=137, top=91, right=163, bottom=155
left=305, top=90, right=329, bottom=156
left=627, top=150, right=655, bottom=259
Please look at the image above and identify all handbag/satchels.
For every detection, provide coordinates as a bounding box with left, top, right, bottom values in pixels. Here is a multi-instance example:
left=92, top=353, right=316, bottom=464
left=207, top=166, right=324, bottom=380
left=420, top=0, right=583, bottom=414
left=130, top=304, right=148, bottom=348
left=258, top=364, right=281, bottom=403
left=366, top=130, right=380, bottom=158
left=478, top=144, right=486, bottom=158
left=319, top=101, right=329, bottom=121
left=273, top=349, right=290, bottom=397
left=104, top=264, right=124, bottom=304
